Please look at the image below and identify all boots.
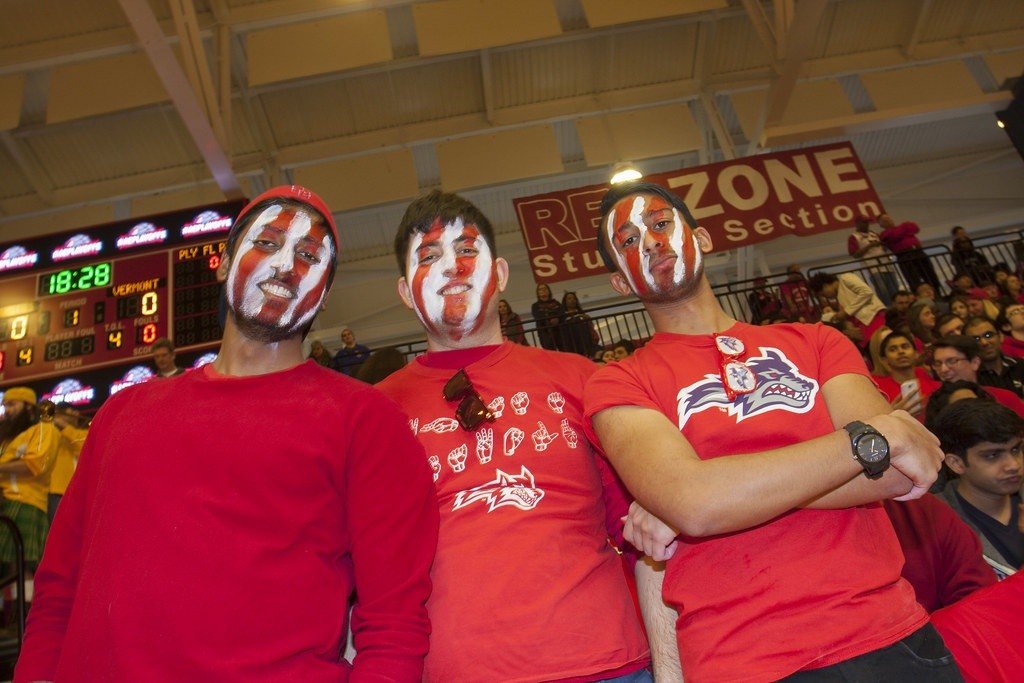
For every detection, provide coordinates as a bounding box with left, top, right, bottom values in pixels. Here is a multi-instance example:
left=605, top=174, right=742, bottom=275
left=0, top=601, right=34, bottom=638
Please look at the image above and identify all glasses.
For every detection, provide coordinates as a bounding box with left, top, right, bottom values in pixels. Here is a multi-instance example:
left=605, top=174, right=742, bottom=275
left=931, top=357, right=968, bottom=370
left=1007, top=308, right=1024, bottom=319
left=712, top=332, right=757, bottom=402
left=442, top=368, right=495, bottom=431
left=974, top=332, right=998, bottom=342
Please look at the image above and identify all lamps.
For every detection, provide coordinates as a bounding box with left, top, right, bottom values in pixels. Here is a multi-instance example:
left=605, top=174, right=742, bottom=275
left=609, top=161, right=642, bottom=184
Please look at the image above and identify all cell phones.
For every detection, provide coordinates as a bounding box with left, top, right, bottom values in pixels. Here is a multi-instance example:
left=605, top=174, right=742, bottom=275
left=900, top=379, right=921, bottom=416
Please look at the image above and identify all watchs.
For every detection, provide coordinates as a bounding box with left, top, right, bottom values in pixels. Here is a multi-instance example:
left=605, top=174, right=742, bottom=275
left=843, top=420, right=892, bottom=480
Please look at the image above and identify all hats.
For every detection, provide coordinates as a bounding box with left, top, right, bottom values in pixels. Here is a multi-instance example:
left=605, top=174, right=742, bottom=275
left=227, top=185, right=341, bottom=257
left=2, top=386, right=37, bottom=406
left=788, top=264, right=800, bottom=273
left=753, top=276, right=767, bottom=284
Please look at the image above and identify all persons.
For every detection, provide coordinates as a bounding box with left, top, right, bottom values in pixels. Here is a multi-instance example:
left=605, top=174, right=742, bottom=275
left=365, top=189, right=685, bottom=683
left=147, top=338, right=187, bottom=379
left=333, top=328, right=368, bottom=375
left=742, top=263, right=1023, bottom=611
left=0, top=386, right=92, bottom=615
left=358, top=348, right=406, bottom=386
left=847, top=215, right=901, bottom=299
left=577, top=179, right=969, bottom=682
left=498, top=300, right=530, bottom=347
left=531, top=282, right=638, bottom=366
left=309, top=341, right=335, bottom=371
left=874, top=214, right=943, bottom=297
left=14, top=183, right=437, bottom=683
left=950, top=225, right=987, bottom=277
